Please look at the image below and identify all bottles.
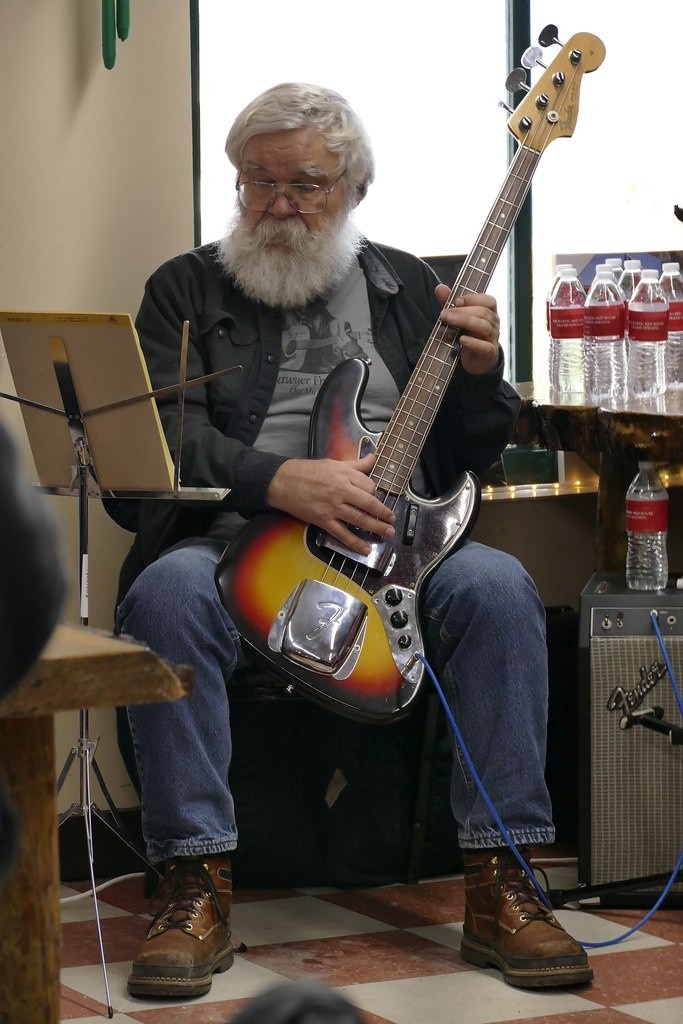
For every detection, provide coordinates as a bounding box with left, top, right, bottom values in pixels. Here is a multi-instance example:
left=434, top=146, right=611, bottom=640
left=627, top=462, right=669, bottom=591
left=546, top=258, right=683, bottom=408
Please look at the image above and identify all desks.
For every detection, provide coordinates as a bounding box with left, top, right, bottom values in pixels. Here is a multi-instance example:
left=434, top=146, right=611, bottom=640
left=509, top=382, right=683, bottom=574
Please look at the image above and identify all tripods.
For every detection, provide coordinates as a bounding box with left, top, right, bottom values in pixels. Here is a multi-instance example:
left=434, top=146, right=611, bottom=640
left=0, top=321, right=242, bottom=1018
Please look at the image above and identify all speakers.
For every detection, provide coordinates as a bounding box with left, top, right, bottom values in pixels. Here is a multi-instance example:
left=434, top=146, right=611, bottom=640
left=219, top=683, right=440, bottom=887
left=577, top=571, right=683, bottom=909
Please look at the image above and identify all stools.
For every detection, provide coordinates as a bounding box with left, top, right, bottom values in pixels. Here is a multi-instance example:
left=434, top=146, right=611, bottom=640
left=144, top=662, right=443, bottom=899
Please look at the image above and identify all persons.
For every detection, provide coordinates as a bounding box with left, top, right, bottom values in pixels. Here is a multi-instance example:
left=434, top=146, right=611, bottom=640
left=102, top=82, right=592, bottom=1002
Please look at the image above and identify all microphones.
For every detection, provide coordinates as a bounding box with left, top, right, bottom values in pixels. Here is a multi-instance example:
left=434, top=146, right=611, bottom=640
left=619, top=708, right=665, bottom=730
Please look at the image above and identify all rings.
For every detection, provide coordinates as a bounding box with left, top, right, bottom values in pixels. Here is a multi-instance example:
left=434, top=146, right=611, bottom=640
left=484, top=327, right=495, bottom=341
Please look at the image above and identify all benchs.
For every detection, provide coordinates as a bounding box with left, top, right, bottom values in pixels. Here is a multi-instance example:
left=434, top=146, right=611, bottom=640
left=1, top=623, right=184, bottom=1023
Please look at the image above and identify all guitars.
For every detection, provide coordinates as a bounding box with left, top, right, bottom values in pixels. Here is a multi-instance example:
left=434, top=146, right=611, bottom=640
left=213, top=20, right=607, bottom=722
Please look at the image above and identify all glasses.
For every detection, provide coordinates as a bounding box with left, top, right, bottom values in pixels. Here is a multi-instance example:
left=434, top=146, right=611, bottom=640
left=237, top=168, right=355, bottom=215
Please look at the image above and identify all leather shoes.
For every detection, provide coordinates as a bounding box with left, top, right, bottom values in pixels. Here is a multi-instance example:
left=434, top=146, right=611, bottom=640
left=460, top=855, right=599, bottom=989
left=123, top=855, right=235, bottom=1002
